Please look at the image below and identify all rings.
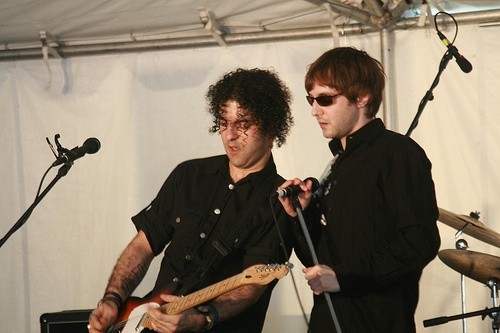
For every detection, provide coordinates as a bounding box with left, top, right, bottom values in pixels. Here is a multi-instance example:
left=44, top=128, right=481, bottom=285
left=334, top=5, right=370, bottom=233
left=87, top=324, right=91, bottom=329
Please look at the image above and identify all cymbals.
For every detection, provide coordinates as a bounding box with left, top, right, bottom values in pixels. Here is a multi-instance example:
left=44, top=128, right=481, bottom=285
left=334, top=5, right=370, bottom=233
left=437, top=249, right=500, bottom=291
left=436, top=206, right=500, bottom=247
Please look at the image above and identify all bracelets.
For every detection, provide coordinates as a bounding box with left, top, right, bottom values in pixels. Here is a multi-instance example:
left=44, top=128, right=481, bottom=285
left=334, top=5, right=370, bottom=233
left=96, top=291, right=124, bottom=309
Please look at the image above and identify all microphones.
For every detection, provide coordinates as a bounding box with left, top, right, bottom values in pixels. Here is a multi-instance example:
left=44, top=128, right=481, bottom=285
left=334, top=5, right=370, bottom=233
left=53, top=138, right=101, bottom=165
left=437, top=31, right=472, bottom=73
left=276, top=177, right=320, bottom=197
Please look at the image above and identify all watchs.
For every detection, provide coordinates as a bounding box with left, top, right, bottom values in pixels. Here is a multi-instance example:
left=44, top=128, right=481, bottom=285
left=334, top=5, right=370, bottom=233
left=196, top=305, right=214, bottom=331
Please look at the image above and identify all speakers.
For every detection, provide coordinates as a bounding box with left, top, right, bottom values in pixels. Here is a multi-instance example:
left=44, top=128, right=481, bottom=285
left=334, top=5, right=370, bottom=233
left=39, top=309, right=96, bottom=333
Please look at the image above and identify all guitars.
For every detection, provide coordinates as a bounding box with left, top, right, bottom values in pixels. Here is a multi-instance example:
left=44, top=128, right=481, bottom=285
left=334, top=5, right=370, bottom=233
left=99, top=260, right=294, bottom=333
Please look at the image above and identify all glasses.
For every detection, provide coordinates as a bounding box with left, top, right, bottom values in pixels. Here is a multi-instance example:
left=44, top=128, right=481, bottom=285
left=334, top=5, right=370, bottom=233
left=305, top=91, right=344, bottom=106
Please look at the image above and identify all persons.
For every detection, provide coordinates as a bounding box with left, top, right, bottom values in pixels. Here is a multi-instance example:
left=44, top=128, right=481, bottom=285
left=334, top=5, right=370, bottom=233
left=87, top=67, right=294, bottom=333
left=278, top=47, right=441, bottom=333
